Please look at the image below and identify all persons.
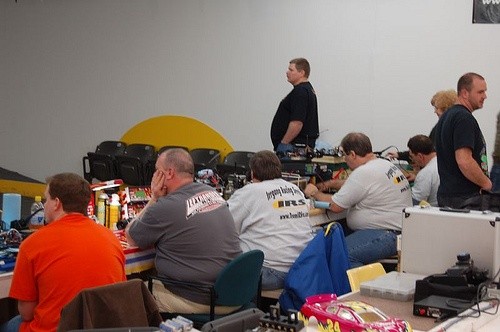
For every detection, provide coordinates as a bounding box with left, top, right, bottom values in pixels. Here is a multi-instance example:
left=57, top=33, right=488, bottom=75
left=1, top=171, right=128, bottom=331
left=269, top=57, right=320, bottom=161
left=488, top=111, right=500, bottom=211
left=304, top=132, right=415, bottom=269
left=125, top=149, right=244, bottom=332
left=386, top=89, right=459, bottom=161
left=435, top=72, right=492, bottom=210
left=398, top=135, right=441, bottom=206
left=224, top=149, right=317, bottom=292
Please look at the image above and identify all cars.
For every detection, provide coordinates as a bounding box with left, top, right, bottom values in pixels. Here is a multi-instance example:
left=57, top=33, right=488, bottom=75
left=300, top=294, right=412, bottom=332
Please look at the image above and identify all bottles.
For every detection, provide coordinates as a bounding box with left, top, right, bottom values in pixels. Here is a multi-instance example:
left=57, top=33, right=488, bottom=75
left=224, top=181, right=234, bottom=199
left=109, top=195, right=121, bottom=228
left=98, top=193, right=110, bottom=229
left=30, top=196, right=45, bottom=229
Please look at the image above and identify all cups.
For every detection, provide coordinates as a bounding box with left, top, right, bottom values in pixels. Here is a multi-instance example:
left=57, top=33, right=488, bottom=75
left=2, top=193, right=22, bottom=229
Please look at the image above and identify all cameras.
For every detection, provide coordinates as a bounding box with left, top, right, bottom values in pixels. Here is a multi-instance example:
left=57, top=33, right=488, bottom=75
left=117, top=218, right=133, bottom=230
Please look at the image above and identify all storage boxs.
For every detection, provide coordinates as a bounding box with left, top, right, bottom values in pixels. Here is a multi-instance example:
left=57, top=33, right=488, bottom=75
left=399, top=204, right=500, bottom=283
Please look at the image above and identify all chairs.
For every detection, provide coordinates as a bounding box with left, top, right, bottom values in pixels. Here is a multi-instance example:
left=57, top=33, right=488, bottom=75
left=346, top=262, right=386, bottom=292
left=83, top=141, right=255, bottom=185
left=262, top=222, right=341, bottom=304
left=147, top=249, right=265, bottom=328
left=79, top=278, right=149, bottom=332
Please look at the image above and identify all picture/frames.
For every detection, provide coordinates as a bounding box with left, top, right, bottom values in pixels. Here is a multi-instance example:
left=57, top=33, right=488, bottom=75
left=472, top=0, right=500, bottom=24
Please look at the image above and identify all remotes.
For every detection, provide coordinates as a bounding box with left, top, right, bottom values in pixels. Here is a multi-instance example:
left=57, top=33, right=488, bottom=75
left=440, top=208, right=470, bottom=213
